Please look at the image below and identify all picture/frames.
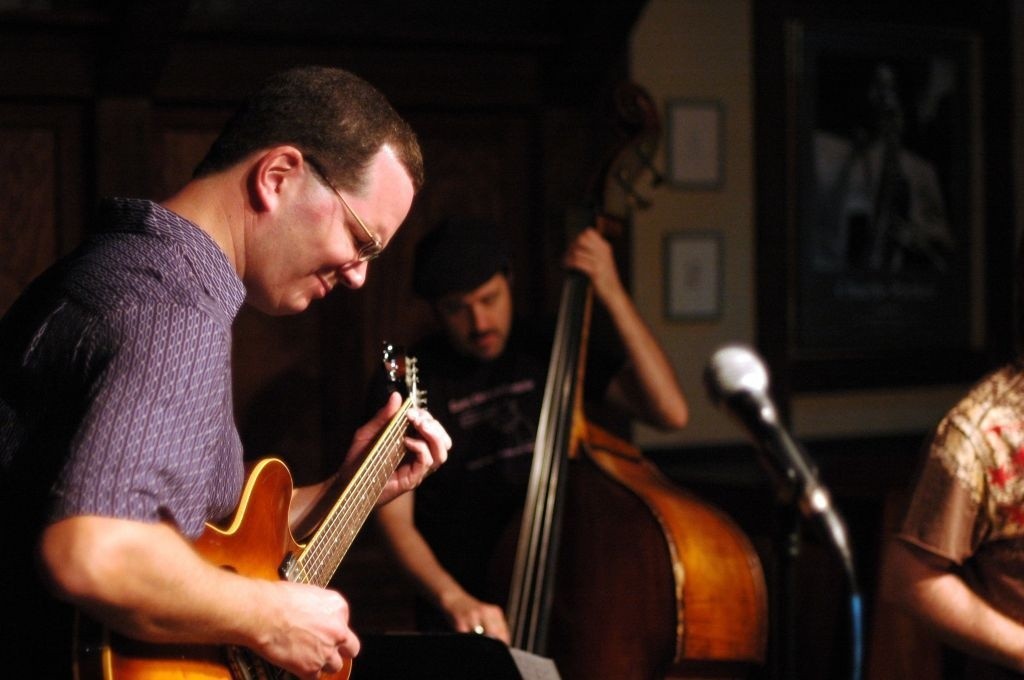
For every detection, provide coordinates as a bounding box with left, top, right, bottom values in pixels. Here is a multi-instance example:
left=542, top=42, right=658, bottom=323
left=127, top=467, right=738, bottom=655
left=662, top=229, right=722, bottom=321
left=663, top=98, right=725, bottom=190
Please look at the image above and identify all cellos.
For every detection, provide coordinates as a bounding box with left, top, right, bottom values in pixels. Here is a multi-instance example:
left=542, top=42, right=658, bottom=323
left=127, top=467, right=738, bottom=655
left=478, top=79, right=771, bottom=680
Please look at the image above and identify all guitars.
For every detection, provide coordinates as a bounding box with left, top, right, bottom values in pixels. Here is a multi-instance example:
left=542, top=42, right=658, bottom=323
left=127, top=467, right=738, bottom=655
left=71, top=339, right=429, bottom=680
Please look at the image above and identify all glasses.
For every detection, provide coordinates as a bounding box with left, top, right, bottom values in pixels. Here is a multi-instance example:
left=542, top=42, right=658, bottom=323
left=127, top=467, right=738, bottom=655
left=300, top=155, right=382, bottom=262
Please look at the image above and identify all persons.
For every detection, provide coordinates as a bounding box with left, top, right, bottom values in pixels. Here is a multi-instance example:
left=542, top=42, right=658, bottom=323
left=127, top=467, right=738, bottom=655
left=0, top=67, right=451, bottom=680
left=878, top=347, right=1024, bottom=680
left=390, top=216, right=690, bottom=644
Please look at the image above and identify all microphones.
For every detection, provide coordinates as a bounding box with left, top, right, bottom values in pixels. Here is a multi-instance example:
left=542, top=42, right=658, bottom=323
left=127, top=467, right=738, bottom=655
left=703, top=344, right=847, bottom=552
